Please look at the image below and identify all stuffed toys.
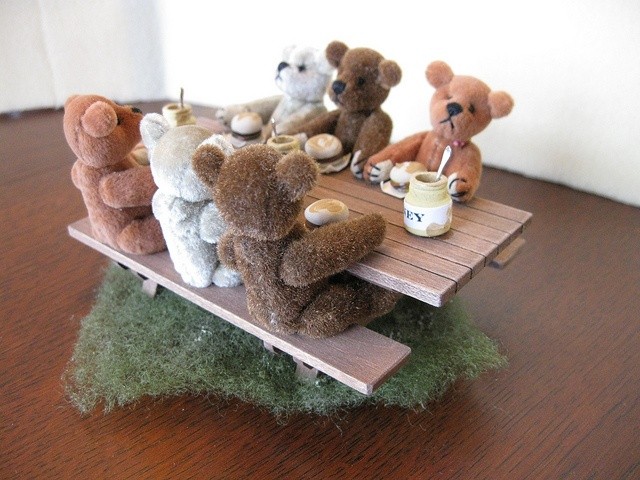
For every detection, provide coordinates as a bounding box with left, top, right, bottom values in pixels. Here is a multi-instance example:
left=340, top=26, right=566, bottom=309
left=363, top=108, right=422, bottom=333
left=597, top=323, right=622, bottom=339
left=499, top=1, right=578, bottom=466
left=63, top=94, right=166, bottom=255
left=191, top=142, right=404, bottom=340
left=216, top=44, right=336, bottom=138
left=139, top=111, right=244, bottom=289
left=277, top=40, right=403, bottom=180
left=362, top=60, right=514, bottom=203
